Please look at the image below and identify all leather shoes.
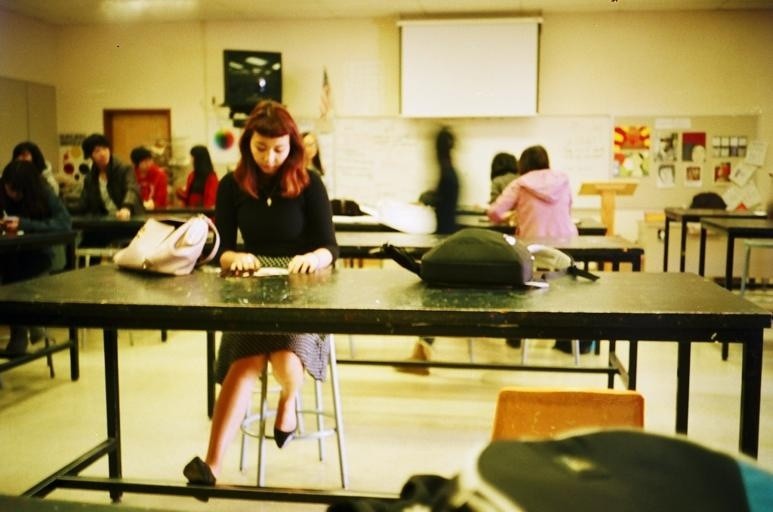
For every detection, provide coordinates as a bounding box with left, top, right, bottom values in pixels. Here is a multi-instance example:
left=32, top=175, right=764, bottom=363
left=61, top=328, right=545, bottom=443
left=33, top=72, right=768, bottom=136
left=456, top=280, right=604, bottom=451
left=274, top=393, right=299, bottom=449
left=184, top=456, right=216, bottom=503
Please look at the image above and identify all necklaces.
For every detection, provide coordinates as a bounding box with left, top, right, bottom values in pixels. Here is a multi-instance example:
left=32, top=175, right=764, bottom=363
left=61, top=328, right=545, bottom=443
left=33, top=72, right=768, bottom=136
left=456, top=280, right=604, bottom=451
left=266, top=182, right=281, bottom=207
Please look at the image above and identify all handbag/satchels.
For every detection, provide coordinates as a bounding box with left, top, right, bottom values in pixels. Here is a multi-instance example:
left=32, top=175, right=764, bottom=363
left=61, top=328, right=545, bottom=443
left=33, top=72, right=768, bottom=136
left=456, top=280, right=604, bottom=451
left=418, top=227, right=538, bottom=287
left=111, top=210, right=221, bottom=277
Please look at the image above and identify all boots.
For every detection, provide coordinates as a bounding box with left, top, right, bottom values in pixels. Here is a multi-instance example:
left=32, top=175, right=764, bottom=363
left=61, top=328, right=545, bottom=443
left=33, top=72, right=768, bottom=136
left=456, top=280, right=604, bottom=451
left=394, top=342, right=431, bottom=374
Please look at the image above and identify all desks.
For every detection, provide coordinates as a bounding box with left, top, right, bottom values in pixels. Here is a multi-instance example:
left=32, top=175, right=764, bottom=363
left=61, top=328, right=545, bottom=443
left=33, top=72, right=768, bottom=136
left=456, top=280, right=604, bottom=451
left=0, top=253, right=773, bottom=512
left=0, top=200, right=643, bottom=390
left=664, top=205, right=773, bottom=360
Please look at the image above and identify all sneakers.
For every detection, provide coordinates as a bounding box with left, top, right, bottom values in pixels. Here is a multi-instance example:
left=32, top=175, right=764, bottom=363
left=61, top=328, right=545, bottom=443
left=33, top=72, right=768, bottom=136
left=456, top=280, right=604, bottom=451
left=4, top=324, right=48, bottom=359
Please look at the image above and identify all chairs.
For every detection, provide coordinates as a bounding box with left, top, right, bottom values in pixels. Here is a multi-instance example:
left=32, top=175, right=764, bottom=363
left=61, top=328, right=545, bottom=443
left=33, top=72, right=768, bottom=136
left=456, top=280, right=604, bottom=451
left=239, top=328, right=351, bottom=490
left=468, top=431, right=773, bottom=512
left=488, top=389, right=644, bottom=441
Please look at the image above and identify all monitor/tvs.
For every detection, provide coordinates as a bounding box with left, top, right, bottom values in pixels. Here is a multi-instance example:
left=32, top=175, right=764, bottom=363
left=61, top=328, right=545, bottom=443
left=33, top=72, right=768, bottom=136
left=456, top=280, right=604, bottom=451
left=223, top=48, right=282, bottom=105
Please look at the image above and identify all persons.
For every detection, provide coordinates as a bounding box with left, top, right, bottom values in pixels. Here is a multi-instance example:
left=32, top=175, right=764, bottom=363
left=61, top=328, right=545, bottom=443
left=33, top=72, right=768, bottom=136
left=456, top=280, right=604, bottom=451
left=489, top=152, right=520, bottom=205
left=78, top=133, right=146, bottom=269
left=13, top=141, right=60, bottom=198
left=130, top=146, right=169, bottom=211
left=486, top=145, right=580, bottom=348
left=299, top=132, right=325, bottom=177
left=175, top=146, right=220, bottom=231
left=0, top=160, right=73, bottom=359
left=396, top=127, right=460, bottom=377
left=184, top=99, right=339, bottom=503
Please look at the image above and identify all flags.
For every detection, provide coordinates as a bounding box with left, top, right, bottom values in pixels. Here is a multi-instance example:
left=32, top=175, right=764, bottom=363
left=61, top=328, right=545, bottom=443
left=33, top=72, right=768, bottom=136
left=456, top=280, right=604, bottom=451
left=314, top=71, right=333, bottom=123
